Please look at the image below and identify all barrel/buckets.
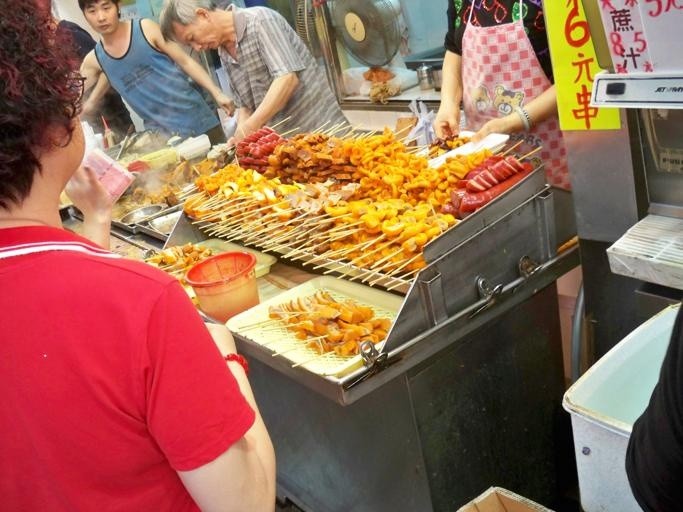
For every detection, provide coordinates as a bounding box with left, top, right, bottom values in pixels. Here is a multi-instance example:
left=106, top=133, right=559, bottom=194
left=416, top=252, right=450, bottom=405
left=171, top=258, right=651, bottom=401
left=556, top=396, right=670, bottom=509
left=183, top=251, right=259, bottom=323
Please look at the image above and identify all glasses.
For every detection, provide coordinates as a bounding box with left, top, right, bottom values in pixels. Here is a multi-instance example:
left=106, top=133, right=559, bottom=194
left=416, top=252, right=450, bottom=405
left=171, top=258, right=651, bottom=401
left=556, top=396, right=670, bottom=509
left=60, top=70, right=87, bottom=103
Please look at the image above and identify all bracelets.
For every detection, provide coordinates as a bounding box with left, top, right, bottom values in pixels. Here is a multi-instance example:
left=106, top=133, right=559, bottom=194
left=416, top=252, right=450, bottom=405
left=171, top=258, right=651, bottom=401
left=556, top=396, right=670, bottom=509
left=515, top=107, right=532, bottom=134
left=224, top=352, right=249, bottom=376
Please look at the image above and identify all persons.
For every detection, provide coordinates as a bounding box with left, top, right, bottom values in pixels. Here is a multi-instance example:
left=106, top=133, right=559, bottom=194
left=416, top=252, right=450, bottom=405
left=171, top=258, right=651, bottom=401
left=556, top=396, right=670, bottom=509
left=433, top=0, right=572, bottom=192
left=625, top=297, right=683, bottom=512
left=47, top=18, right=137, bottom=148
left=0, top=0, right=277, bottom=512
left=78, top=0, right=236, bottom=150
left=158, top=0, right=355, bottom=148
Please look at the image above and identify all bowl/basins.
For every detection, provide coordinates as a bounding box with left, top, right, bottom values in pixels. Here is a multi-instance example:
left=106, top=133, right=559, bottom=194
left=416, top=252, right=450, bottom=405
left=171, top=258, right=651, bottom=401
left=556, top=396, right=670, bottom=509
left=120, top=205, right=163, bottom=225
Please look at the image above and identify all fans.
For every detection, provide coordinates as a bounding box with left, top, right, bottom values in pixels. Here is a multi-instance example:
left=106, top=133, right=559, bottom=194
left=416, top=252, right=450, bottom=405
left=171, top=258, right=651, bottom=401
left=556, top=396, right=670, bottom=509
left=328, top=0, right=418, bottom=94
left=294, top=0, right=323, bottom=59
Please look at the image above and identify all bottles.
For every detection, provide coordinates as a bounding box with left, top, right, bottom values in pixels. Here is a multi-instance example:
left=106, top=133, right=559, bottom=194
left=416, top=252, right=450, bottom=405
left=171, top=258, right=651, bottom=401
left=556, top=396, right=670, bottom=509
left=417, top=63, right=433, bottom=89
left=432, top=66, right=442, bottom=91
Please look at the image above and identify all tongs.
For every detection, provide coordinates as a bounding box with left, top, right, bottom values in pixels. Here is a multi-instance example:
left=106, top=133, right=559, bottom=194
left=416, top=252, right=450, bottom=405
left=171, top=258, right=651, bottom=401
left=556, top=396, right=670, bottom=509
left=216, top=146, right=237, bottom=169
